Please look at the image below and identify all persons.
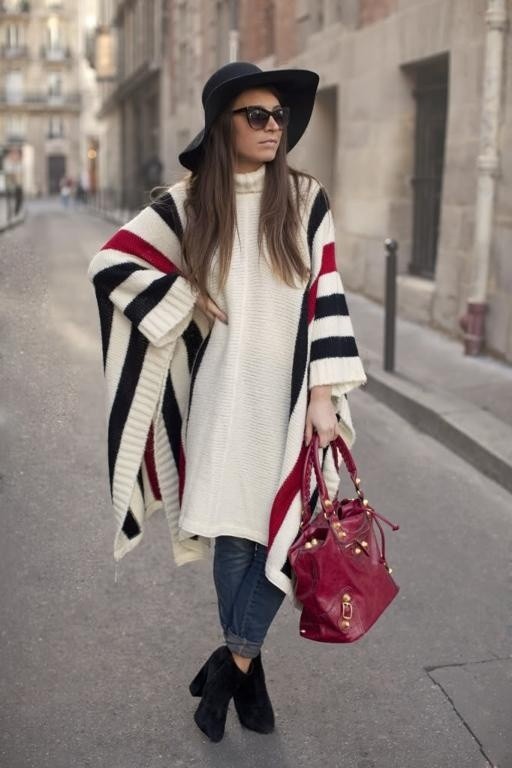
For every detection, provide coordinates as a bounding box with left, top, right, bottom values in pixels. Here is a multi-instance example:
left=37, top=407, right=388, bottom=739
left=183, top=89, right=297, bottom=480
left=89, top=62, right=368, bottom=742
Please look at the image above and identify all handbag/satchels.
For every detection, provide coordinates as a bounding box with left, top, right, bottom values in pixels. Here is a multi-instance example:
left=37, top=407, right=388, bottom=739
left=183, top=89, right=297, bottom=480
left=287, top=430, right=401, bottom=645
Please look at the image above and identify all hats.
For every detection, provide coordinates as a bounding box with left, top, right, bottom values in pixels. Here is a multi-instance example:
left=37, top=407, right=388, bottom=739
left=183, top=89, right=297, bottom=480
left=178, top=61, right=320, bottom=172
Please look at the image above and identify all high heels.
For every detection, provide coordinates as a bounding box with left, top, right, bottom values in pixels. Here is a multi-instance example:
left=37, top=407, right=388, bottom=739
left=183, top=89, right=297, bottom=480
left=190, top=645, right=276, bottom=743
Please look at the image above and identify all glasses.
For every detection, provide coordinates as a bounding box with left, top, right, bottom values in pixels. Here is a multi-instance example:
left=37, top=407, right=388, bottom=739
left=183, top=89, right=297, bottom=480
left=232, top=106, right=290, bottom=129
